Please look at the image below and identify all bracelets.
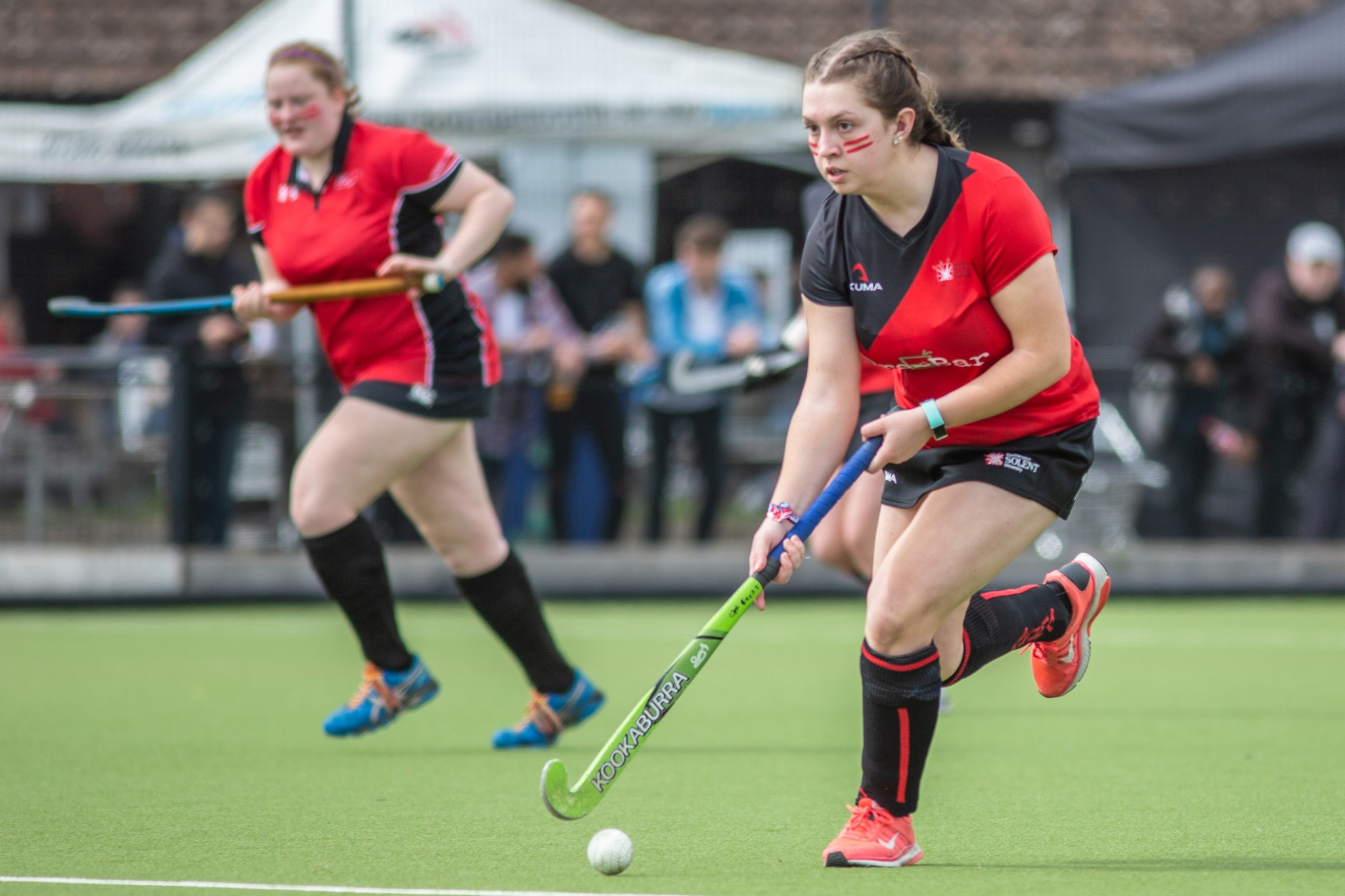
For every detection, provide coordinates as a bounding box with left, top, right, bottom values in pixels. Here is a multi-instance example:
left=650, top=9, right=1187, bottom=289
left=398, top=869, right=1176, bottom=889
left=765, top=502, right=801, bottom=524
left=919, top=398, right=948, bottom=441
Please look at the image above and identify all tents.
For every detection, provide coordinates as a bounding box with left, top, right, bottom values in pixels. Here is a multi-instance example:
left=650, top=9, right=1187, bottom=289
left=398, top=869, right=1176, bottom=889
left=1056, top=0, right=1345, bottom=415
left=0, top=0, right=810, bottom=459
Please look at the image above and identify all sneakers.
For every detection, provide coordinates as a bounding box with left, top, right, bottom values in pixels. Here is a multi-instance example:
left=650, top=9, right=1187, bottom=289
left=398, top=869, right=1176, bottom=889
left=1018, top=552, right=1112, bottom=698
left=489, top=668, right=607, bottom=748
left=822, top=797, right=923, bottom=868
left=323, top=653, right=441, bottom=737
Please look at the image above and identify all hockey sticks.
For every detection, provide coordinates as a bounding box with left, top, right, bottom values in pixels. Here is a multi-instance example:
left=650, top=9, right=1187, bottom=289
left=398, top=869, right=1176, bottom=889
left=540, top=404, right=904, bottom=822
left=48, top=274, right=444, bottom=318
left=667, top=347, right=802, bottom=398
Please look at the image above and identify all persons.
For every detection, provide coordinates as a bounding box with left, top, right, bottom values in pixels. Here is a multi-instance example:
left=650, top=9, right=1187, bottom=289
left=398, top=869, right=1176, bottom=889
left=230, top=40, right=604, bottom=752
left=749, top=32, right=1111, bottom=870
left=148, top=182, right=1345, bottom=583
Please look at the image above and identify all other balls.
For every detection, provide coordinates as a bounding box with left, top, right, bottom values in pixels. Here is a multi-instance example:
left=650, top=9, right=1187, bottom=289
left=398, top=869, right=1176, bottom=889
left=585, top=827, right=634, bottom=876
